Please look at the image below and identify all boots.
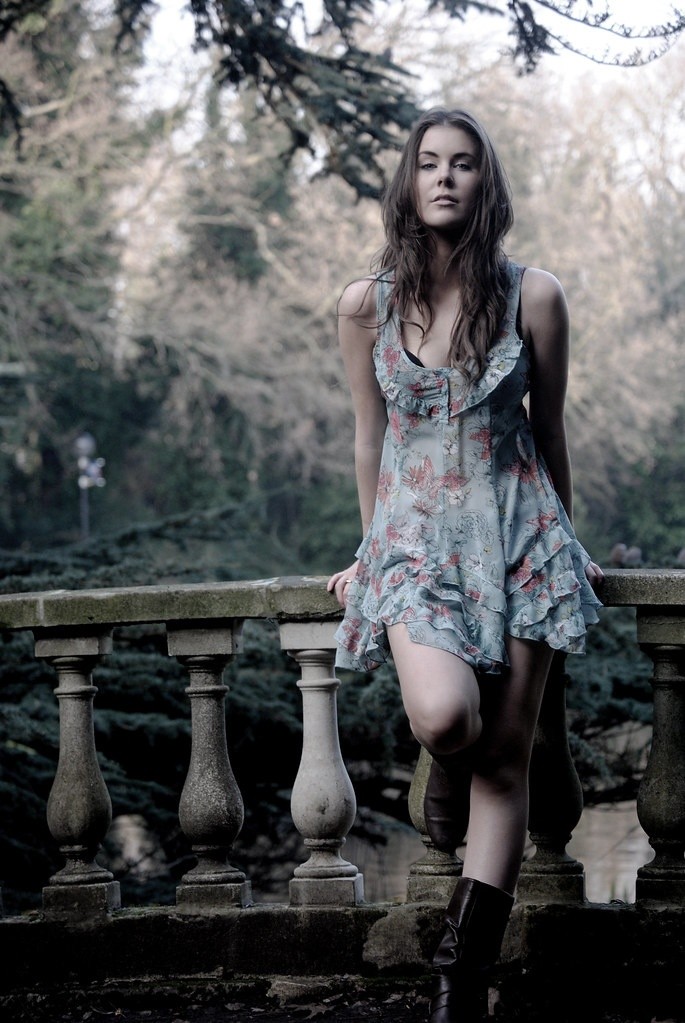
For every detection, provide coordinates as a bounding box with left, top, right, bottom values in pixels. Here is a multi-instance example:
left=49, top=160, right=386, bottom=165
left=425, top=748, right=475, bottom=852
left=427, top=876, right=515, bottom=1023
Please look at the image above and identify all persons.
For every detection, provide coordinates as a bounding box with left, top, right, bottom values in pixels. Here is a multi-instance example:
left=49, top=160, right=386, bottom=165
left=328, top=110, right=606, bottom=1023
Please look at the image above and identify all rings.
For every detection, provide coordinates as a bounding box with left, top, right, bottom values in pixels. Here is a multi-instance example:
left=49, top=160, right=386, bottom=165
left=345, top=579, right=352, bottom=583
